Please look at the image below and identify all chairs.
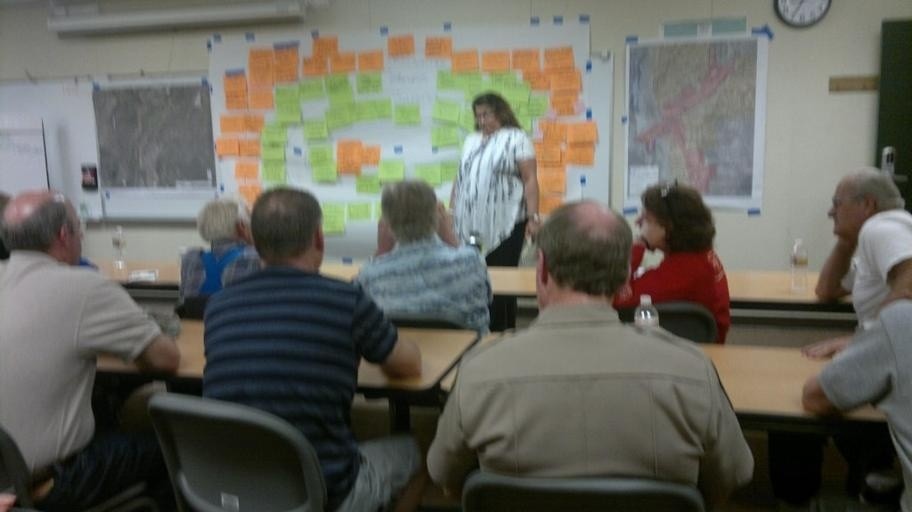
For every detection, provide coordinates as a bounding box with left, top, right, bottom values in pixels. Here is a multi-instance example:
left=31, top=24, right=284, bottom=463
left=2, top=430, right=165, bottom=512
left=150, top=392, right=328, bottom=511
left=461, top=472, right=703, bottom=511
left=614, top=304, right=716, bottom=344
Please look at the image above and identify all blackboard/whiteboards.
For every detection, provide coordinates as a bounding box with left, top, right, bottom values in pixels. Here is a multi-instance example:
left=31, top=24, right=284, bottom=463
left=2, top=51, right=613, bottom=229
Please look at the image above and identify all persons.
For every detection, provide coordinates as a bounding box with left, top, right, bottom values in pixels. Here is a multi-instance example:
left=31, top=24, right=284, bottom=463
left=202, top=188, right=428, bottom=512
left=349, top=178, right=494, bottom=349
left=611, top=179, right=731, bottom=345
left=447, top=93, right=541, bottom=333
left=800, top=300, right=911, bottom=512
left=178, top=197, right=260, bottom=321
left=800, top=166, right=912, bottom=361
left=425, top=199, right=755, bottom=512
left=0, top=189, right=178, bottom=512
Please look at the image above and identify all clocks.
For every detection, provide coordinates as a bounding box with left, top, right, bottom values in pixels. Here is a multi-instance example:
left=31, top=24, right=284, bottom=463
left=774, top=1, right=831, bottom=29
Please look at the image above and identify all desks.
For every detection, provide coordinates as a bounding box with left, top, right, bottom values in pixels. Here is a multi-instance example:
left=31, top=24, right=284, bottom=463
left=94, top=319, right=479, bottom=438
left=87, top=258, right=859, bottom=333
left=440, top=329, right=886, bottom=511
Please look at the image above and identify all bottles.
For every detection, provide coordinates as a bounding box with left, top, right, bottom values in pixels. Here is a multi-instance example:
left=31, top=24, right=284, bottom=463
left=112, top=224, right=128, bottom=270
left=468, top=235, right=479, bottom=249
left=789, top=237, right=808, bottom=291
left=634, top=294, right=660, bottom=327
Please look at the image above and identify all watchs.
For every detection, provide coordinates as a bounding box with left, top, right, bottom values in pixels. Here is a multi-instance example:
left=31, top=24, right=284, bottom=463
left=527, top=212, right=540, bottom=225
